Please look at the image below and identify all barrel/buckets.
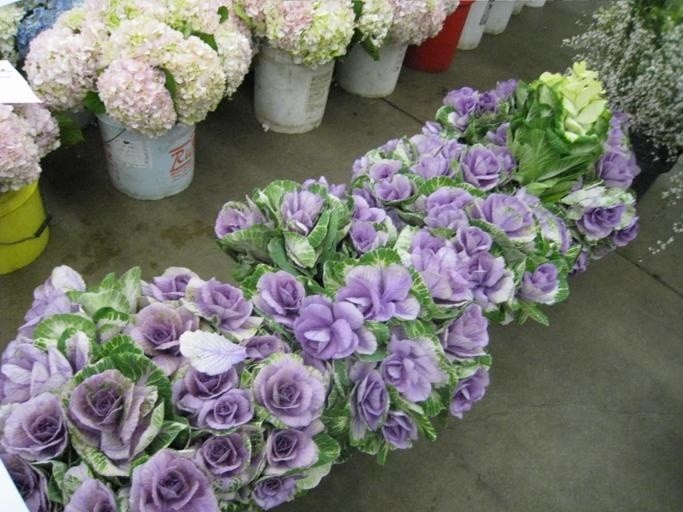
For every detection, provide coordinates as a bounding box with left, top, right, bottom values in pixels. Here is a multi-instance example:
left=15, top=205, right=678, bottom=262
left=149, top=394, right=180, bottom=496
left=0, top=180, right=50, bottom=275
left=407, top=0, right=474, bottom=72
left=525, top=0, right=546, bottom=8
left=337, top=38, right=408, bottom=98
left=96, top=114, right=195, bottom=200
left=253, top=38, right=336, bottom=133
left=485, top=0, right=514, bottom=35
left=457, top=0, right=495, bottom=50
left=512, top=0, right=526, bottom=16
